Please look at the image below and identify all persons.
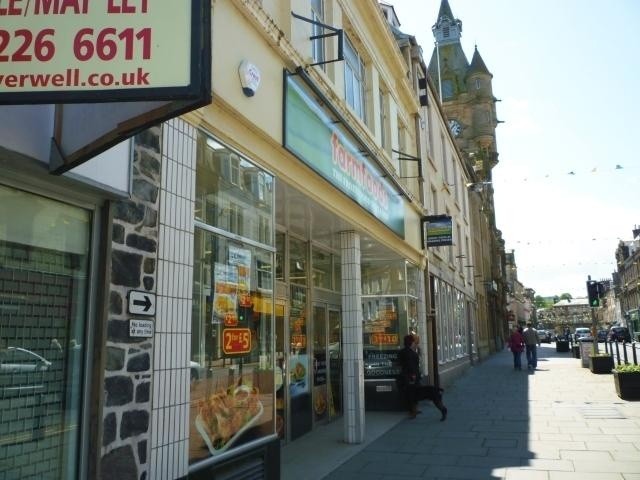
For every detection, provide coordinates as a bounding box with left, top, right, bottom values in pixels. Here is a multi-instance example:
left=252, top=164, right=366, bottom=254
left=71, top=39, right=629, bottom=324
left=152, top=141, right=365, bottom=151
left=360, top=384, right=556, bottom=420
left=522, top=323, right=541, bottom=370
left=508, top=327, right=524, bottom=371
left=410, top=334, right=423, bottom=356
left=398, top=334, right=421, bottom=419
left=564, top=324, right=570, bottom=340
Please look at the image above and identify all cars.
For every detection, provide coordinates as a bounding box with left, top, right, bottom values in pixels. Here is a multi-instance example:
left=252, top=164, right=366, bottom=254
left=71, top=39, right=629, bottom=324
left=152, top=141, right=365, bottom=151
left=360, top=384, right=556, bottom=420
left=0, top=347, right=54, bottom=401
left=536, top=326, right=630, bottom=343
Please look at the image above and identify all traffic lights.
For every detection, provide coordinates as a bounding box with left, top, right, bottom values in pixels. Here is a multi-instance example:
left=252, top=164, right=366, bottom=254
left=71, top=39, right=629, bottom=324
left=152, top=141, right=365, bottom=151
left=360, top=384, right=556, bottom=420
left=587, top=280, right=600, bottom=307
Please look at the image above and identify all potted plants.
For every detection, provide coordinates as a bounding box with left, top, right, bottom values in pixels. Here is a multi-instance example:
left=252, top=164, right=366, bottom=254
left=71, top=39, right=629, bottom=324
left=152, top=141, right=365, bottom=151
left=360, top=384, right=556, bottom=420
left=588, top=351, right=640, bottom=401
